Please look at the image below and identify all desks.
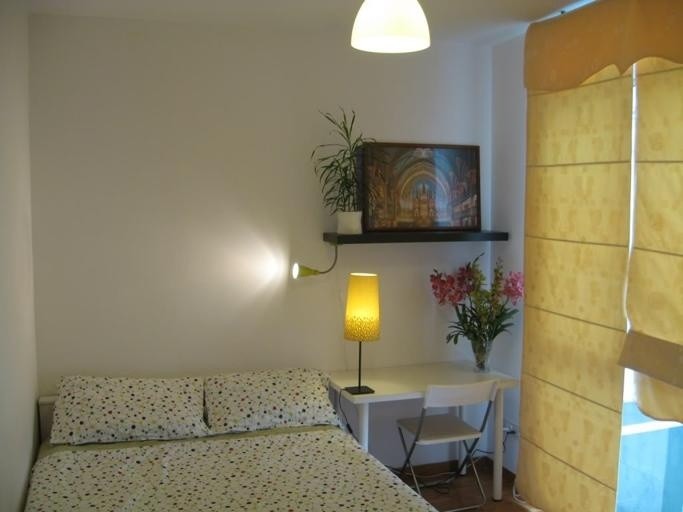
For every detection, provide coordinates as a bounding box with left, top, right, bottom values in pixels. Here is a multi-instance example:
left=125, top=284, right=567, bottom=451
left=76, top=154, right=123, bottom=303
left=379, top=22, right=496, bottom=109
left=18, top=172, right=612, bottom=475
left=325, top=359, right=521, bottom=503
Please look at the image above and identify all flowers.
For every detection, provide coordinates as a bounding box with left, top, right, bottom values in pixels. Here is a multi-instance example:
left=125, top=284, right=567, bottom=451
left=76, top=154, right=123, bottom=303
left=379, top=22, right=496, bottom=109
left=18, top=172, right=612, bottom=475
left=428, top=251, right=524, bottom=367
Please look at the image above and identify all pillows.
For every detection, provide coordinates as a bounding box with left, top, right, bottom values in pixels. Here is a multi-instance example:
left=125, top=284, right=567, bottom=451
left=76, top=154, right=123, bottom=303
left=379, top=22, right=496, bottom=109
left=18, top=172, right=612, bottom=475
left=48, top=370, right=206, bottom=445
left=199, top=363, right=346, bottom=434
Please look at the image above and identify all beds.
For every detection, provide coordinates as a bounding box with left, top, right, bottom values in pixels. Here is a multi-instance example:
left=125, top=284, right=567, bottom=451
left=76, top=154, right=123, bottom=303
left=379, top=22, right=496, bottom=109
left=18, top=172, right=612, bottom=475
left=25, top=360, right=443, bottom=512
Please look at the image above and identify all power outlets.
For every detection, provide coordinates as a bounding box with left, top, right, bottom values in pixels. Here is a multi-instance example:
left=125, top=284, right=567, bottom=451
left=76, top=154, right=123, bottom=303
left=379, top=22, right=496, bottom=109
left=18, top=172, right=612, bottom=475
left=502, top=419, right=519, bottom=439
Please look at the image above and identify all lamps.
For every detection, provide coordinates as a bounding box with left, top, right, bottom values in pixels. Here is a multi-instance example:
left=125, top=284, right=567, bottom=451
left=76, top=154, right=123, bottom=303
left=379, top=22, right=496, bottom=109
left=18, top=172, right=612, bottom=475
left=340, top=270, right=381, bottom=397
left=287, top=235, right=340, bottom=282
left=346, top=1, right=434, bottom=57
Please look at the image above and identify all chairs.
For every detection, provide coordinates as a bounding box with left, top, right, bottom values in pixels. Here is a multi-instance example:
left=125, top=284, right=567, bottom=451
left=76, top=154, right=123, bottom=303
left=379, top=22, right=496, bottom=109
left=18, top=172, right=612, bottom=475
left=395, top=378, right=499, bottom=511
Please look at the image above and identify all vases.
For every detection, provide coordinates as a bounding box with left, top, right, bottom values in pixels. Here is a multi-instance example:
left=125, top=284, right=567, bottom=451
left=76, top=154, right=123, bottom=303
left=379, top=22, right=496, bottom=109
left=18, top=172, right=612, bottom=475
left=471, top=337, right=492, bottom=371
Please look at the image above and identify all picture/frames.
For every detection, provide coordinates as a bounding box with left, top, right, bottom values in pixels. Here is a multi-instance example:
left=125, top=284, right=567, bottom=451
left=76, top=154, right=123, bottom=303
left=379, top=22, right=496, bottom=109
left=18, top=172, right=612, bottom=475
left=356, top=138, right=483, bottom=234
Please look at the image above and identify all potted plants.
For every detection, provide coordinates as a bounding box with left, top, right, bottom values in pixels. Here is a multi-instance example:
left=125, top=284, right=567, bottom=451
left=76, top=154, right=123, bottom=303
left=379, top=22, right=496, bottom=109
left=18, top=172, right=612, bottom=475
left=308, top=104, right=368, bottom=232
